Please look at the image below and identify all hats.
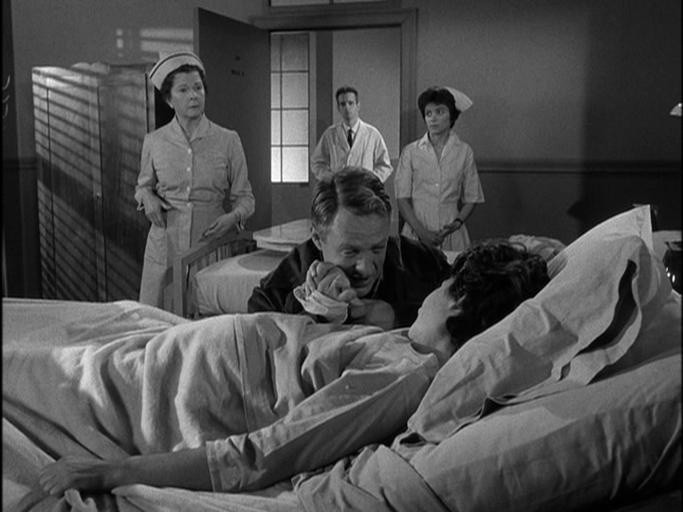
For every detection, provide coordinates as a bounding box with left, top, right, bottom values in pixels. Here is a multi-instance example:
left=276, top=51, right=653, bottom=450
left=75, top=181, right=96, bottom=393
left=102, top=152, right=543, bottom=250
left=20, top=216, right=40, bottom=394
left=149, top=52, right=206, bottom=91
left=445, top=87, right=473, bottom=112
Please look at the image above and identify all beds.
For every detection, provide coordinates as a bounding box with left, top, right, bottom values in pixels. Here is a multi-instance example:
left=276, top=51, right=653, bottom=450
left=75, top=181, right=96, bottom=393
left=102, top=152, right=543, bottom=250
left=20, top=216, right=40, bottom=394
left=173, top=230, right=681, bottom=345
left=1, top=292, right=680, bottom=512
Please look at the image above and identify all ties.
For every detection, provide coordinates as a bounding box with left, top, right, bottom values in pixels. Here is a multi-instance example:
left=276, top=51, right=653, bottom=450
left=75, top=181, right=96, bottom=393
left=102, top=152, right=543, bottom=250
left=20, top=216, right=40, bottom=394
left=348, top=129, right=352, bottom=148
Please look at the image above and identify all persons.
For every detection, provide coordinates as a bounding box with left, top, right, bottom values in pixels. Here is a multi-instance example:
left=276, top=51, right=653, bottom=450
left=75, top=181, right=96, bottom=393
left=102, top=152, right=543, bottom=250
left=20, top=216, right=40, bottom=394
left=246, top=165, right=453, bottom=330
left=309, top=86, right=394, bottom=185
left=134, top=50, right=256, bottom=322
left=392, top=85, right=486, bottom=266
left=0, top=240, right=549, bottom=502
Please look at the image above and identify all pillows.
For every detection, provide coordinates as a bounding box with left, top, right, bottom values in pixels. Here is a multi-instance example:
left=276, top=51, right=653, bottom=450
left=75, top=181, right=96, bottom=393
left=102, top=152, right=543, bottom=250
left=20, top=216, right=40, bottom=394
left=405, top=203, right=660, bottom=448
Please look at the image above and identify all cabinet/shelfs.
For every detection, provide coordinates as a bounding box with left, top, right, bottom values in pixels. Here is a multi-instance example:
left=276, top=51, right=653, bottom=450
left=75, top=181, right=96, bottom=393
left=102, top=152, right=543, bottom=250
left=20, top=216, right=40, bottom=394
left=30, top=62, right=174, bottom=304
